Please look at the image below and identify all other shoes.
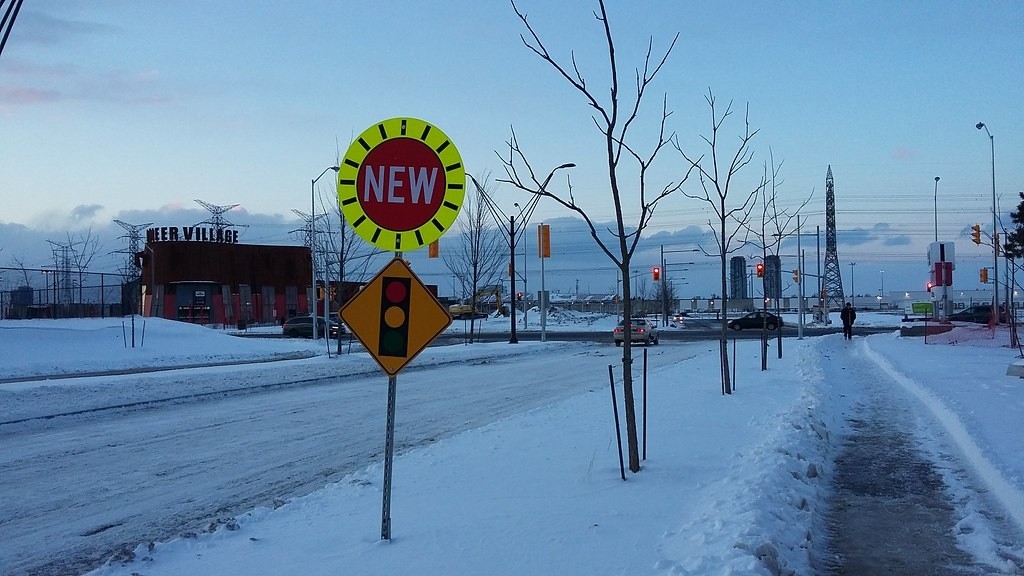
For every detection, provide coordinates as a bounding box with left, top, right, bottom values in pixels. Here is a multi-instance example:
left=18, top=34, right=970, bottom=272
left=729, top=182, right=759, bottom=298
left=849, top=338, right=852, bottom=341
left=844, top=335, right=847, bottom=340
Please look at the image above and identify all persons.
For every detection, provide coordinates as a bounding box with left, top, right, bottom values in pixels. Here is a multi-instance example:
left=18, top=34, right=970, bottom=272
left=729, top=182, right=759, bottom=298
left=840, top=302, right=856, bottom=340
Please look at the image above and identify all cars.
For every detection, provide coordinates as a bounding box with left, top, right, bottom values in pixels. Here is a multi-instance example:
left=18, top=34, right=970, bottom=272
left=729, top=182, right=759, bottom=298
left=726, top=311, right=784, bottom=331
left=613, top=318, right=660, bottom=347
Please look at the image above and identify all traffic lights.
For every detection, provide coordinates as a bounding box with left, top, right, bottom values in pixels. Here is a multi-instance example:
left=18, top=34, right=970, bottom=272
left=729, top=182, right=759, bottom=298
left=518, top=293, right=522, bottom=301
left=792, top=269, right=798, bottom=283
left=653, top=267, right=660, bottom=281
left=757, top=263, right=764, bottom=278
left=926, top=282, right=932, bottom=292
left=971, top=224, right=980, bottom=245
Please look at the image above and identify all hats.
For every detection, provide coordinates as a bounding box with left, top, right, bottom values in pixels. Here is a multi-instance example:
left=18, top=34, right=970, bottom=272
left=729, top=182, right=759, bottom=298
left=846, top=302, right=851, bottom=306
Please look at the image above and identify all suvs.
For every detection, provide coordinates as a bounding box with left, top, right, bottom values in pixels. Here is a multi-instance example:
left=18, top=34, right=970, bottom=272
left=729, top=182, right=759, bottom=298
left=283, top=315, right=347, bottom=340
left=946, top=304, right=1010, bottom=324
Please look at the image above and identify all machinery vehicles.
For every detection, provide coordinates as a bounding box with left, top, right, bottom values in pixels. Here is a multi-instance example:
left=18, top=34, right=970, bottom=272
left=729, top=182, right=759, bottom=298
left=448, top=287, right=510, bottom=321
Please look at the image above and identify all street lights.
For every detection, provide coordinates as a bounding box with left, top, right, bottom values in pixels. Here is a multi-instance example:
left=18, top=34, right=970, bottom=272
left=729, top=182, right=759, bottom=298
left=311, top=165, right=342, bottom=340
left=880, top=270, right=884, bottom=297
left=464, top=162, right=577, bottom=345
left=663, top=258, right=694, bottom=293
left=513, top=202, right=527, bottom=330
left=934, top=175, right=942, bottom=241
left=975, top=122, right=1000, bottom=326
left=771, top=225, right=822, bottom=324
left=661, top=244, right=701, bottom=327
